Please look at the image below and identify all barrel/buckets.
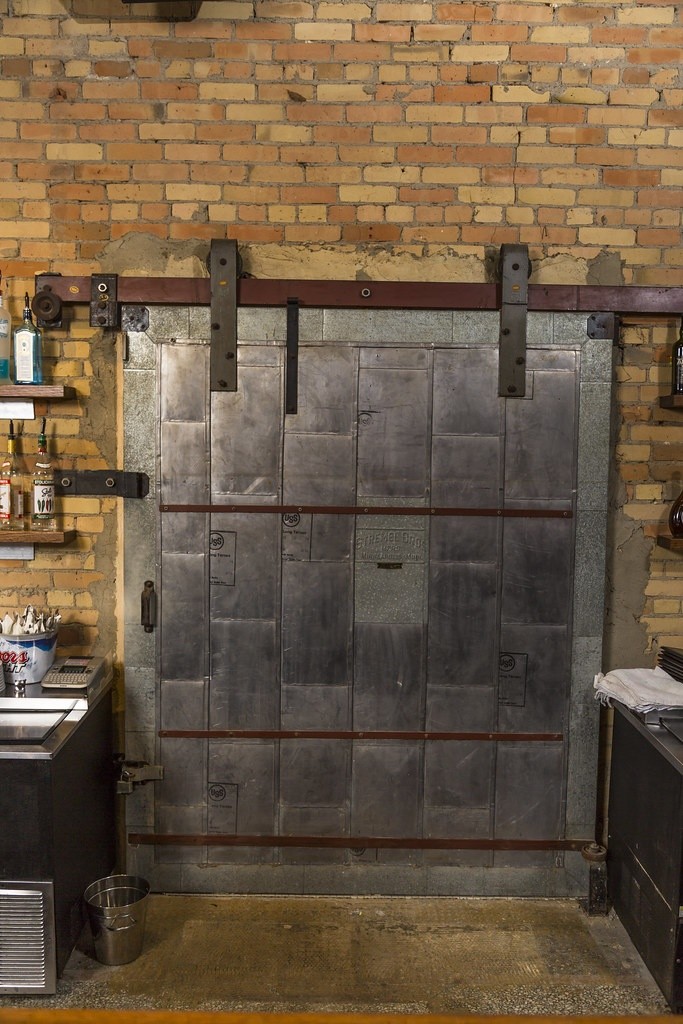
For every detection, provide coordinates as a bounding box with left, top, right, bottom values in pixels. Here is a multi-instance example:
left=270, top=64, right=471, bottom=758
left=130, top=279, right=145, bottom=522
left=0, top=628, right=60, bottom=686
left=84, top=874, right=151, bottom=967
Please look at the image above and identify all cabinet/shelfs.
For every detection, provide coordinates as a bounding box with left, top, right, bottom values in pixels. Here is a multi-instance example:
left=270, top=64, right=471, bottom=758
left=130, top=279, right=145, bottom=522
left=0, top=385, right=76, bottom=544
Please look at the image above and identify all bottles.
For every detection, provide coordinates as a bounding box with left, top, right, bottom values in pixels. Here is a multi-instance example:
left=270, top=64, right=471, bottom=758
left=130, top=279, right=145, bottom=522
left=14, top=291, right=43, bottom=385
left=669, top=490, right=683, bottom=540
left=29, top=418, right=57, bottom=532
left=0, top=290, right=14, bottom=386
left=0, top=417, right=26, bottom=533
left=671, top=316, right=683, bottom=396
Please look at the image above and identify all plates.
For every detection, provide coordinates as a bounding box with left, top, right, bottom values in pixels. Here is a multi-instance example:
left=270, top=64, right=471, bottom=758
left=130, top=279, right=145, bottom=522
left=0, top=659, right=6, bottom=694
left=660, top=715, right=683, bottom=744
left=657, top=645, right=683, bottom=683
